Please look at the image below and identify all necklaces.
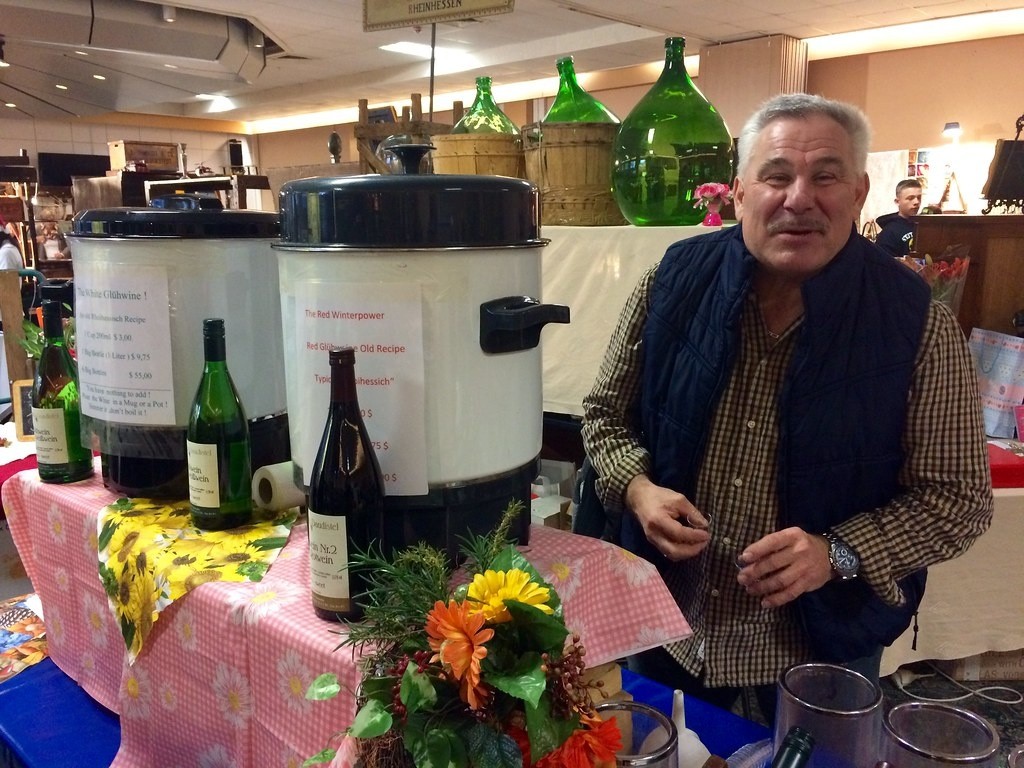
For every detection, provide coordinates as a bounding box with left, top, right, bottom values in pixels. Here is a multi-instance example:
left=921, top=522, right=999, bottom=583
left=768, top=311, right=804, bottom=340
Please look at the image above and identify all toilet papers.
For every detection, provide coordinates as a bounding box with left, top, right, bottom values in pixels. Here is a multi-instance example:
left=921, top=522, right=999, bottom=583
left=251, top=460, right=307, bottom=512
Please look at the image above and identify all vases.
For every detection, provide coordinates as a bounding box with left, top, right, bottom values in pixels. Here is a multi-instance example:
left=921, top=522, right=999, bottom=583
left=702, top=201, right=723, bottom=227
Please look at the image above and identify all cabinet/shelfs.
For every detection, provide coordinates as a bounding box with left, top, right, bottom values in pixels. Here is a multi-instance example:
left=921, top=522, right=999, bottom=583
left=908, top=213, right=1024, bottom=341
left=19, top=185, right=76, bottom=280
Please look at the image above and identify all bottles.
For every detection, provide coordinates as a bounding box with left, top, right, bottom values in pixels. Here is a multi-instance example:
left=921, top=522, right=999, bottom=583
left=535, top=56, right=620, bottom=141
left=609, top=36, right=738, bottom=227
left=307, top=347, right=385, bottom=623
left=449, top=76, right=521, bottom=134
left=32, top=301, right=95, bottom=484
left=187, top=318, right=253, bottom=531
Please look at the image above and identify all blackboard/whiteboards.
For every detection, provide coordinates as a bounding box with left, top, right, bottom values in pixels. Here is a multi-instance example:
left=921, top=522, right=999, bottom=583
left=12, top=379, right=36, bottom=443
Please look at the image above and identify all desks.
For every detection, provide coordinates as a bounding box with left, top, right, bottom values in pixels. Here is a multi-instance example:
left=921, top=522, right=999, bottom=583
left=625, top=667, right=774, bottom=767
left=0, top=451, right=694, bottom=768
left=879, top=437, right=1024, bottom=678
left=541, top=219, right=738, bottom=473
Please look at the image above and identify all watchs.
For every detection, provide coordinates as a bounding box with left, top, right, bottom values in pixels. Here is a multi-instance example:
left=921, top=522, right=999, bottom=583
left=823, top=532, right=859, bottom=580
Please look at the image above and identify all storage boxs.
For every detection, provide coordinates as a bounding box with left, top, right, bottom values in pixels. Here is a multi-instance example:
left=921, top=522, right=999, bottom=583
left=934, top=647, right=1024, bottom=681
left=106, top=140, right=187, bottom=172
left=983, top=442, right=1024, bottom=489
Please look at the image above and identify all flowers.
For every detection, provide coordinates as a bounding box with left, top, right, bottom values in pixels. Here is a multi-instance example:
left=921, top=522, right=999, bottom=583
left=692, top=181, right=734, bottom=210
left=303, top=498, right=624, bottom=768
left=894, top=252, right=970, bottom=317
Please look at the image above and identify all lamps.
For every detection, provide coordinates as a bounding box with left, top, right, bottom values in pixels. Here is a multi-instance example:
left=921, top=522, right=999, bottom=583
left=941, top=122, right=963, bottom=138
left=162, top=4, right=176, bottom=23
left=253, top=27, right=264, bottom=48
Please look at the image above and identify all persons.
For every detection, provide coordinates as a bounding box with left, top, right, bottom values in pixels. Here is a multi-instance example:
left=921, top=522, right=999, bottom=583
left=875, top=180, right=922, bottom=257
left=573, top=94, right=995, bottom=728
left=37, top=215, right=71, bottom=260
left=0, top=225, right=23, bottom=292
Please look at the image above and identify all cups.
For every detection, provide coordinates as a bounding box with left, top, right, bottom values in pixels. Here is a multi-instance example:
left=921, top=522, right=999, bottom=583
left=736, top=551, right=760, bottom=591
left=583, top=702, right=678, bottom=767
left=687, top=509, right=712, bottom=543
left=773, top=662, right=1023, bottom=767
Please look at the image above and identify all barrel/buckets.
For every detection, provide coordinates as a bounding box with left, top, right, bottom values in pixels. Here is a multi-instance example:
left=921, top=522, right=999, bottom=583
left=431, top=120, right=627, bottom=225
